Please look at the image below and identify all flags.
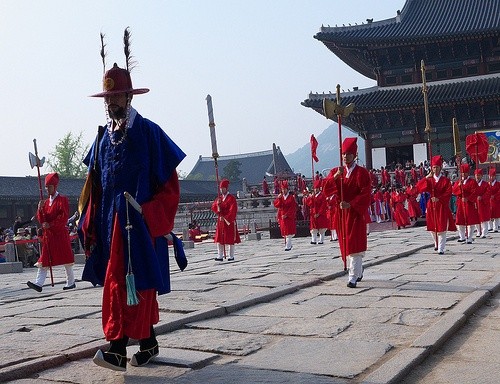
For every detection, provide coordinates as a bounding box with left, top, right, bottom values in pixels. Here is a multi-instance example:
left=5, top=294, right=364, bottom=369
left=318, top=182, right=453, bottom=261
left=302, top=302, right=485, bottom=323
left=311, top=135, right=319, bottom=162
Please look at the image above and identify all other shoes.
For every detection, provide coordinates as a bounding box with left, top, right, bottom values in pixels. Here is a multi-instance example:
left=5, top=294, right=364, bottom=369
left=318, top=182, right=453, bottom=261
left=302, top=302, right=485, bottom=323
left=26, top=281, right=42, bottom=292
left=347, top=281, right=357, bottom=288
left=63, top=284, right=76, bottom=290
left=128, top=340, right=160, bottom=367
left=92, top=349, right=128, bottom=373
left=214, top=257, right=224, bottom=261
left=228, top=257, right=234, bottom=261
left=434, top=248, right=438, bottom=251
left=285, top=239, right=338, bottom=252
left=439, top=251, right=444, bottom=254
left=457, top=229, right=498, bottom=244
left=356, top=275, right=363, bottom=282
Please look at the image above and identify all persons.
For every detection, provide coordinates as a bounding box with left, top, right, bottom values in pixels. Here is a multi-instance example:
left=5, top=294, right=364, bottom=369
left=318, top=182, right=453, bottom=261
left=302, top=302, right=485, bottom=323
left=0, top=212, right=79, bottom=243
left=323, top=137, right=372, bottom=288
left=77, top=60, right=188, bottom=372
left=212, top=179, right=242, bottom=262
left=274, top=156, right=500, bottom=251
left=262, top=176, right=267, bottom=194
left=416, top=155, right=452, bottom=255
left=27, top=173, right=76, bottom=292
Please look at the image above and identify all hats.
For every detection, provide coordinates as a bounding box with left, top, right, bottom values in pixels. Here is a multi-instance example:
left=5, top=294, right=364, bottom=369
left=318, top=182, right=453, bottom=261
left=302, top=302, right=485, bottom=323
left=313, top=180, right=322, bottom=189
left=489, top=167, right=496, bottom=176
left=17, top=228, right=26, bottom=234
left=264, top=171, right=322, bottom=179
left=279, top=180, right=288, bottom=189
left=460, top=164, right=471, bottom=173
left=342, top=137, right=360, bottom=157
left=219, top=179, right=230, bottom=188
left=377, top=176, right=422, bottom=189
left=44, top=173, right=59, bottom=184
left=474, top=168, right=484, bottom=175
left=88, top=25, right=152, bottom=97
left=431, top=155, right=442, bottom=166
